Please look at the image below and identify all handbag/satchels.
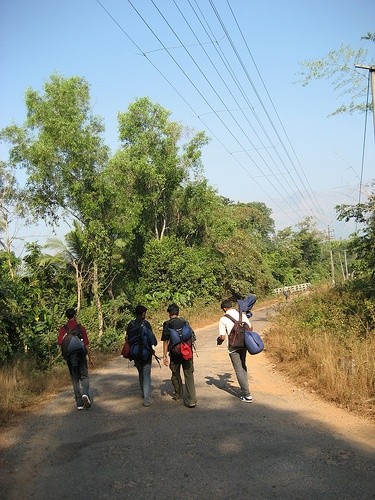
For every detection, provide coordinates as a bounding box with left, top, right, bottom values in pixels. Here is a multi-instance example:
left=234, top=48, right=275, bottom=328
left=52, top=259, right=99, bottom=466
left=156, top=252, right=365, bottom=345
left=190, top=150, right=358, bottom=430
left=236, top=295, right=256, bottom=317
left=181, top=342, right=193, bottom=361
left=167, top=326, right=181, bottom=347
left=180, top=325, right=192, bottom=342
left=244, top=330, right=264, bottom=355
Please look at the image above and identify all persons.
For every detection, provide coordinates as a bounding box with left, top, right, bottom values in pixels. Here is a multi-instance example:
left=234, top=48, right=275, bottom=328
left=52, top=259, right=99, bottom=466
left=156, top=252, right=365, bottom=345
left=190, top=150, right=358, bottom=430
left=284, top=284, right=289, bottom=300
left=161, top=304, right=198, bottom=407
left=123, top=305, right=158, bottom=406
left=216, top=299, right=255, bottom=404
left=57, top=308, right=94, bottom=411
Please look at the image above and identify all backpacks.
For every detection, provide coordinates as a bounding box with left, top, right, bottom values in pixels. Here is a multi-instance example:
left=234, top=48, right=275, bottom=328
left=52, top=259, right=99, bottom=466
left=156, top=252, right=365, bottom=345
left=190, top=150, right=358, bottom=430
left=127, top=320, right=155, bottom=364
left=224, top=309, right=250, bottom=348
left=61, top=324, right=88, bottom=361
left=164, top=316, right=192, bottom=364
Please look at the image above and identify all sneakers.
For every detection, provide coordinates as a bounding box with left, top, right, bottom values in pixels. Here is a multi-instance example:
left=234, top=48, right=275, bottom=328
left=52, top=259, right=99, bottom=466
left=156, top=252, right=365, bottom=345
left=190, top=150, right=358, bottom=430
left=239, top=396, right=253, bottom=403
left=76, top=394, right=91, bottom=410
left=184, top=400, right=196, bottom=407
left=140, top=399, right=151, bottom=406
left=172, top=390, right=183, bottom=401
